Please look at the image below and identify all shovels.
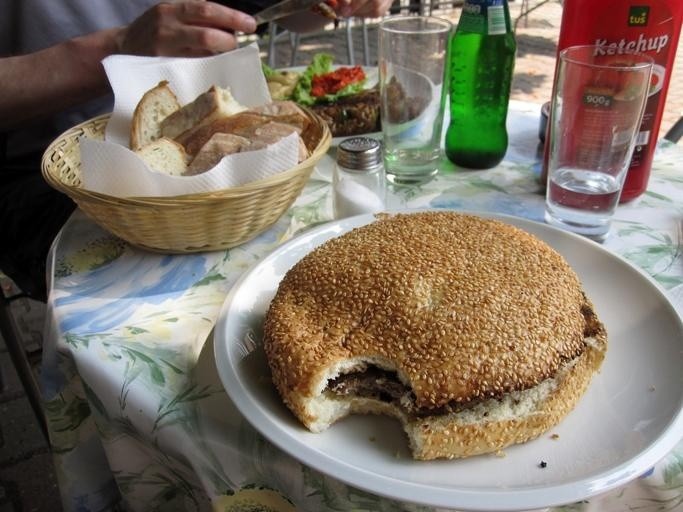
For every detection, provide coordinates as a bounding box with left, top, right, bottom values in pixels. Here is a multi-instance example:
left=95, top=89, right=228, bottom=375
left=265, top=211, right=608, bottom=460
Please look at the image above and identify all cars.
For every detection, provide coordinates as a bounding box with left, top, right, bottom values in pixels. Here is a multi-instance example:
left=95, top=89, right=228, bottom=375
left=376, top=15, right=453, bottom=190
left=543, top=44, right=655, bottom=235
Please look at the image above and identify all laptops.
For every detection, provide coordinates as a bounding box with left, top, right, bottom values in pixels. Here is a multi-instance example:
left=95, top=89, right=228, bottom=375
left=184, top=100, right=313, bottom=151
left=130, top=80, right=180, bottom=149
left=159, top=85, right=241, bottom=140
left=185, top=131, right=249, bottom=177
left=133, top=138, right=188, bottom=179
left=249, top=122, right=308, bottom=165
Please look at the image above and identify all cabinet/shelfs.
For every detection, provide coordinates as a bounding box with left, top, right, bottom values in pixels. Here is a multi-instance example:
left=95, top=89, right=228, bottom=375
left=333, top=138, right=386, bottom=217
left=540, top=0, right=683, bottom=204
left=445, top=0, right=517, bottom=169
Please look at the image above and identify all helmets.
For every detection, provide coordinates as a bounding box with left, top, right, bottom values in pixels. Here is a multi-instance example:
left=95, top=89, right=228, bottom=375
left=267, top=64, right=437, bottom=148
left=211, top=205, right=681, bottom=512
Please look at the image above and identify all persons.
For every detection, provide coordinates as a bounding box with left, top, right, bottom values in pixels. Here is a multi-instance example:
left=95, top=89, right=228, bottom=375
left=1, top=0, right=396, bottom=301
